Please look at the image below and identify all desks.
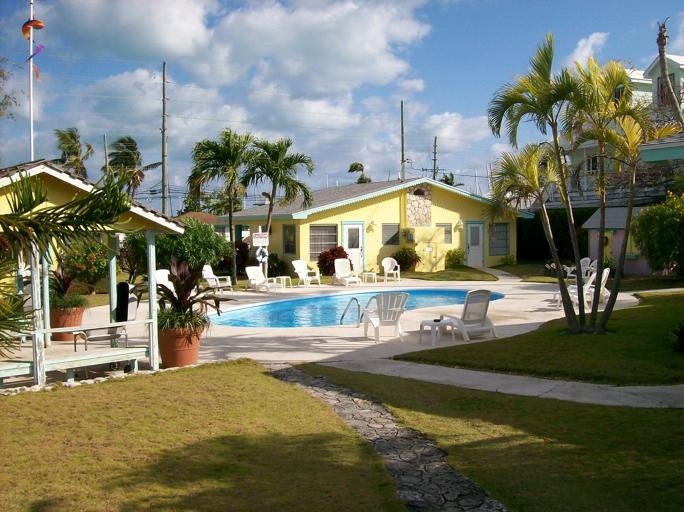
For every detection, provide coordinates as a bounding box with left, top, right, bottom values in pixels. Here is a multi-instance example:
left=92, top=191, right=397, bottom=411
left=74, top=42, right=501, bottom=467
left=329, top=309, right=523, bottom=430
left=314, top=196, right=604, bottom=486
left=363, top=272, right=378, bottom=285
left=276, top=275, right=293, bottom=290
left=418, top=320, right=456, bottom=347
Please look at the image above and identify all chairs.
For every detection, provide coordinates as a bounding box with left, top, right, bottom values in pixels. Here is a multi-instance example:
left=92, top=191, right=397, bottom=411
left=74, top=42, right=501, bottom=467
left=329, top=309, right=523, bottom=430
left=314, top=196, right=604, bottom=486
left=291, top=259, right=321, bottom=287
left=201, top=264, right=235, bottom=297
left=363, top=293, right=409, bottom=344
left=551, top=256, right=612, bottom=310
left=330, top=258, right=361, bottom=287
left=155, top=268, right=203, bottom=301
left=244, top=266, right=278, bottom=296
left=439, top=289, right=500, bottom=342
left=381, top=257, right=401, bottom=284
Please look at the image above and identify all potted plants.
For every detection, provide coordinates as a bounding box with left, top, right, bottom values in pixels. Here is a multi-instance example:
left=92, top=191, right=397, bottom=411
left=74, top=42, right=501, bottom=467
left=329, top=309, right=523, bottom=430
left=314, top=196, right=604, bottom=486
left=142, top=253, right=238, bottom=370
left=48, top=268, right=90, bottom=342
